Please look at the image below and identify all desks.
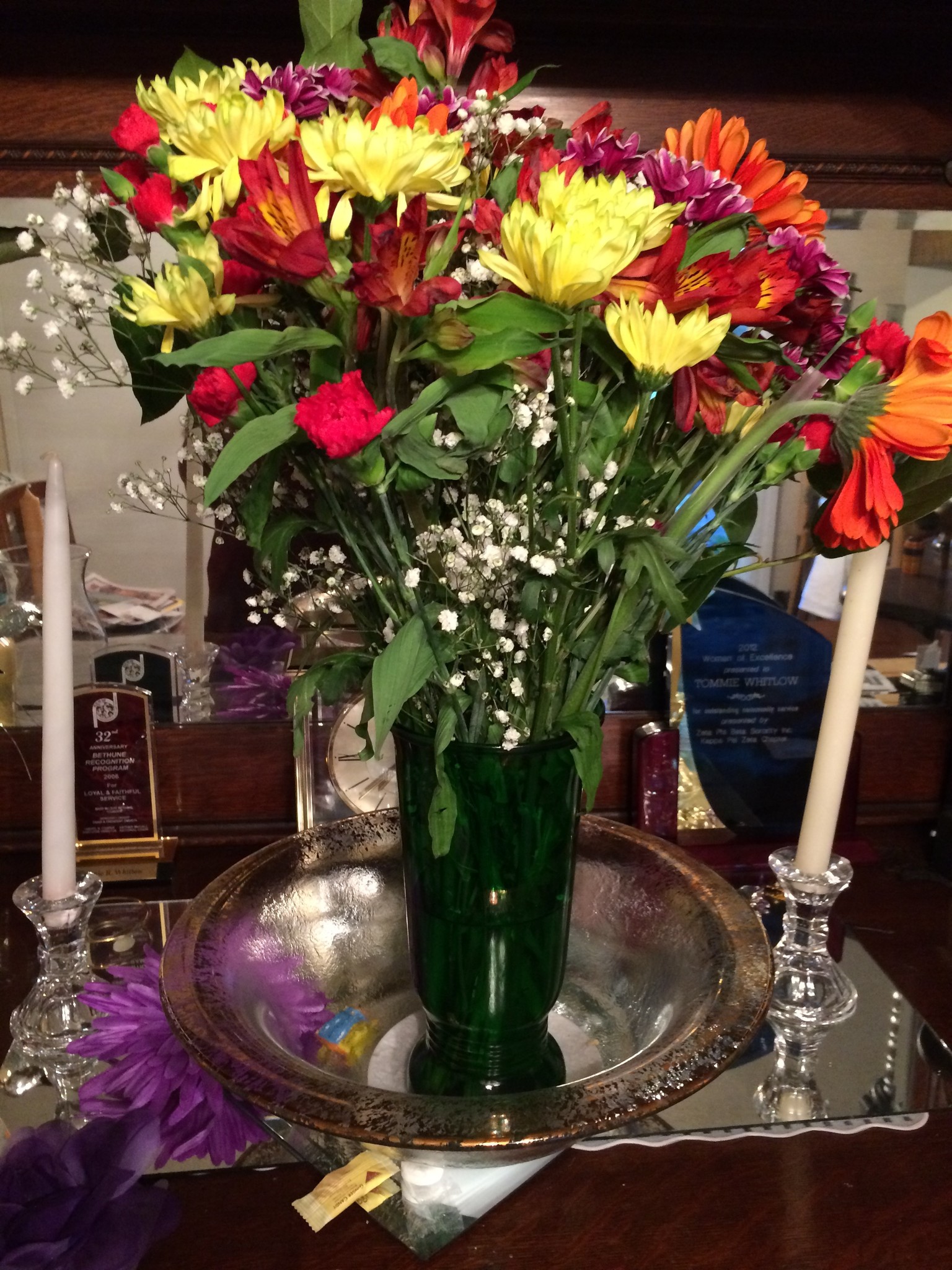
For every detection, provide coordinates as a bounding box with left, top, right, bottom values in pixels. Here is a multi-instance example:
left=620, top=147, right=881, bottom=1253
left=0, top=772, right=950, bottom=1270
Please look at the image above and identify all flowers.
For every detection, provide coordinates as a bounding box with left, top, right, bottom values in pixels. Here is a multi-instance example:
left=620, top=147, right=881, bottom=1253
left=0, top=1117, right=166, bottom=1270
left=76, top=927, right=315, bottom=1186
left=0, top=0, right=951, bottom=746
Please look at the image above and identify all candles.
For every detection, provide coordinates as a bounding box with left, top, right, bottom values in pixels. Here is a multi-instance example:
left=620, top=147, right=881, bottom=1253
left=786, top=524, right=899, bottom=898
left=36, top=446, right=100, bottom=933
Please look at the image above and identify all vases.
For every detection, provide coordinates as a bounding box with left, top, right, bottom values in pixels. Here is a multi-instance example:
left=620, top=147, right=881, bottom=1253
left=377, top=712, right=619, bottom=1113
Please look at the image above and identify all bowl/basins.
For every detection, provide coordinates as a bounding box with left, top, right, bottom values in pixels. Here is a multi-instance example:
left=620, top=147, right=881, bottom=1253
left=157, top=804, right=787, bottom=1148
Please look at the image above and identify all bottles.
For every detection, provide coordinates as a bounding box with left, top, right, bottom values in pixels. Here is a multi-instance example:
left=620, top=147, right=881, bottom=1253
left=0, top=545, right=107, bottom=730
